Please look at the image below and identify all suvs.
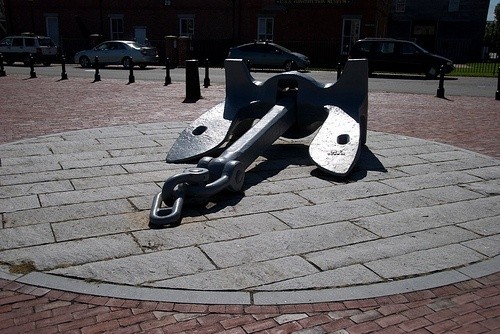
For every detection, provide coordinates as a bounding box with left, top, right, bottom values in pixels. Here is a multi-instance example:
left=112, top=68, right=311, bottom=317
left=0, top=32, right=60, bottom=66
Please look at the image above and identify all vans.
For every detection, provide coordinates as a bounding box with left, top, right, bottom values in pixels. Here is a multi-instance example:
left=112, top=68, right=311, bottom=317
left=348, top=37, right=455, bottom=79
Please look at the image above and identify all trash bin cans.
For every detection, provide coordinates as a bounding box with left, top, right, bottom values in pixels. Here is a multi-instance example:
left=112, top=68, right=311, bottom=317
left=185, top=59, right=201, bottom=99
left=164, top=35, right=178, bottom=68
left=177, top=36, right=190, bottom=67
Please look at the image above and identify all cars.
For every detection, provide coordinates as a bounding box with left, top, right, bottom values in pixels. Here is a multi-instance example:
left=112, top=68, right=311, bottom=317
left=227, top=41, right=310, bottom=72
left=74, top=40, right=159, bottom=70
